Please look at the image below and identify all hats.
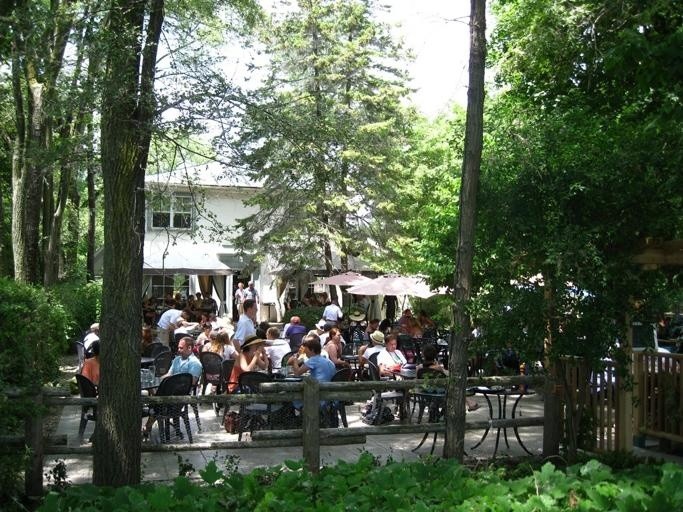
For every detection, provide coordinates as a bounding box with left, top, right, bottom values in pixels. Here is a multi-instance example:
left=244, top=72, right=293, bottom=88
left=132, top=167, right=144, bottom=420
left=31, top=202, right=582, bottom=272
left=370, top=330, right=385, bottom=344
left=242, top=335, right=267, bottom=350
left=315, top=318, right=327, bottom=332
left=349, top=311, right=367, bottom=321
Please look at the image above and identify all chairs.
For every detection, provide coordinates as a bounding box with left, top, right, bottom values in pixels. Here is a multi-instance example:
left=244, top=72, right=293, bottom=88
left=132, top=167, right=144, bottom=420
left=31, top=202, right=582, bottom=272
left=331, top=364, right=353, bottom=428
left=202, top=351, right=235, bottom=424
left=341, top=343, right=360, bottom=371
left=77, top=342, right=87, bottom=370
left=417, top=368, right=448, bottom=424
left=281, top=351, right=298, bottom=369
left=367, top=352, right=410, bottom=424
left=224, top=373, right=301, bottom=440
left=75, top=373, right=99, bottom=437
left=414, top=337, right=449, bottom=366
left=142, top=333, right=203, bottom=445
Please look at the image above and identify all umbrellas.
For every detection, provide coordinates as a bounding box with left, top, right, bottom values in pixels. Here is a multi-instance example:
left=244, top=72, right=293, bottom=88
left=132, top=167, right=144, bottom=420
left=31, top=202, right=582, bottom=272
left=308, top=269, right=454, bottom=317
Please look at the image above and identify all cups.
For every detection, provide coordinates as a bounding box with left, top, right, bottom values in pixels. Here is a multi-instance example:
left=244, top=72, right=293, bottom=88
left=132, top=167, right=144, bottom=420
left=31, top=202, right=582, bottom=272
left=148, top=366, right=157, bottom=385
left=140, top=368, right=149, bottom=383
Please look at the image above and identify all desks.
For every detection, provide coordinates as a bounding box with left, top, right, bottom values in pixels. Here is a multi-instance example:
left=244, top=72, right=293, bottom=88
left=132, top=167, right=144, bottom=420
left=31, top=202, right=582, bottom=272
left=412, top=391, right=475, bottom=456
left=470, top=384, right=535, bottom=458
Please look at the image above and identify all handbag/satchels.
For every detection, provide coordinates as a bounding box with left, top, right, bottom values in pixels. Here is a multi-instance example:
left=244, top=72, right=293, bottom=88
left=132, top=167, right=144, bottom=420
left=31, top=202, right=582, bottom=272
left=363, top=404, right=394, bottom=425
left=225, top=410, right=240, bottom=434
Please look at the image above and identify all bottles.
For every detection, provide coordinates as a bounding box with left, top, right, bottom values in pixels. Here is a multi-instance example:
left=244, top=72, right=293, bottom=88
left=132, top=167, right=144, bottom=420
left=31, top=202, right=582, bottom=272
left=351, top=343, right=358, bottom=357
